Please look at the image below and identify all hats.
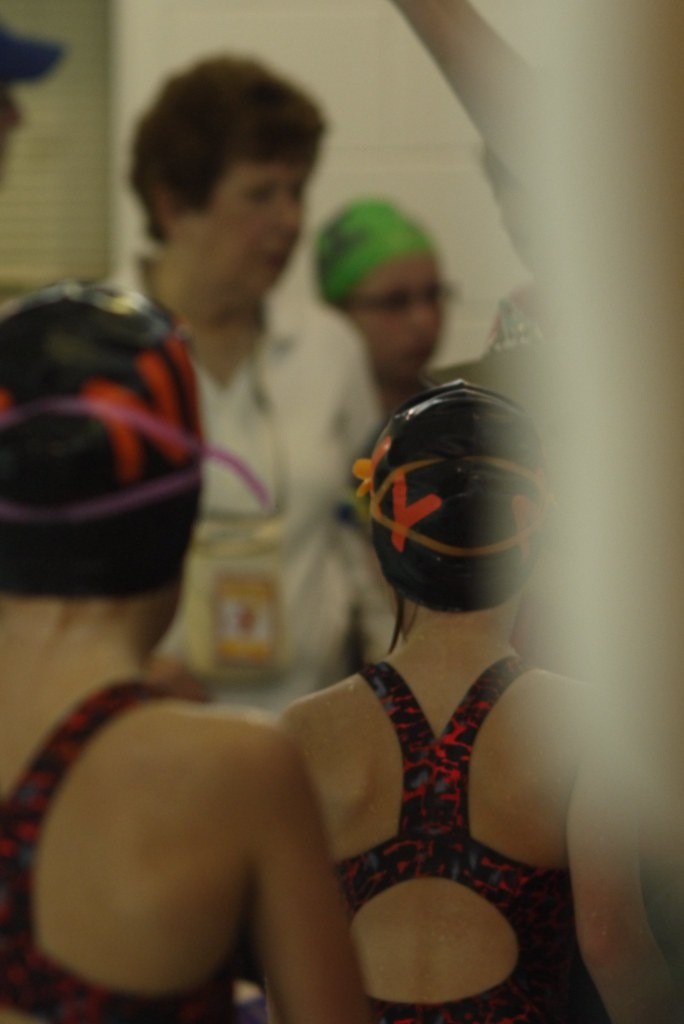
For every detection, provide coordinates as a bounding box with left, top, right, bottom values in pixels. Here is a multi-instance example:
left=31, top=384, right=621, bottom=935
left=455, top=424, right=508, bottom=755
left=352, top=375, right=549, bottom=611
left=313, top=198, right=430, bottom=302
left=0, top=280, right=206, bottom=598
left=0, top=25, right=60, bottom=82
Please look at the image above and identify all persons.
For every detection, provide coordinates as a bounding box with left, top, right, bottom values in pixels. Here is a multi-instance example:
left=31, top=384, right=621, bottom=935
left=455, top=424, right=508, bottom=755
left=312, top=178, right=444, bottom=419
left=261, top=377, right=679, bottom=1024
left=481, top=143, right=559, bottom=355
left=0, top=23, right=62, bottom=194
left=0, top=280, right=367, bottom=1024
left=123, top=56, right=407, bottom=714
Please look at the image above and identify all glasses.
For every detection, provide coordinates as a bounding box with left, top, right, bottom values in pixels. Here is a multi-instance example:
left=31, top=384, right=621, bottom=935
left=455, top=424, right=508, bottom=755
left=372, top=280, right=460, bottom=314
